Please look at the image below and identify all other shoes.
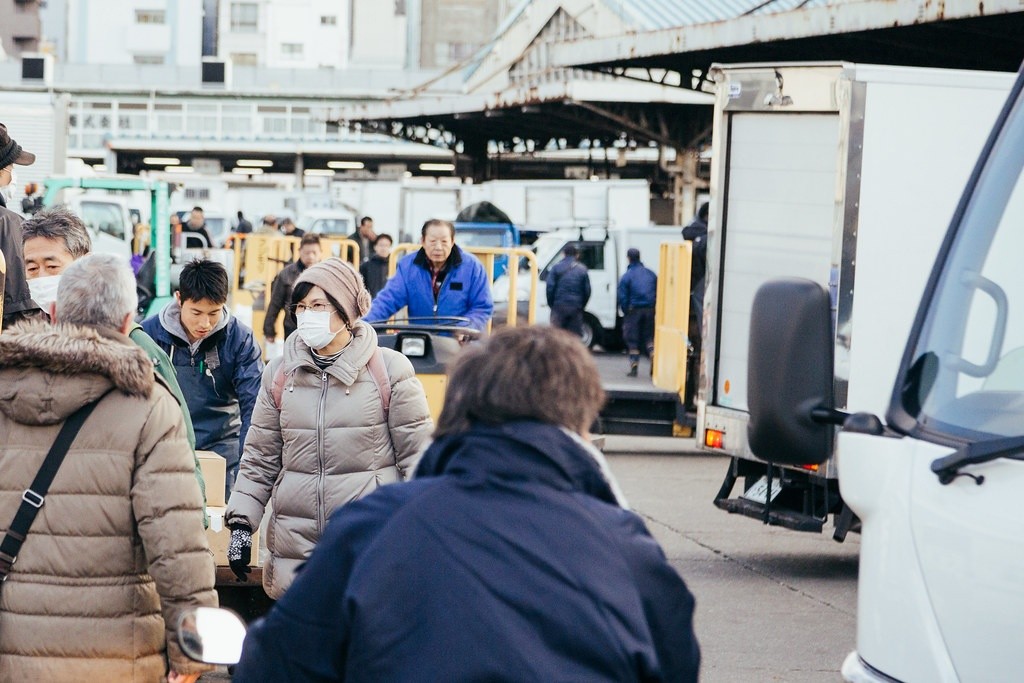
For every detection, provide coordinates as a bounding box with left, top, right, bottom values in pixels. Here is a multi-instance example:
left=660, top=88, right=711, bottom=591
left=649, top=355, right=654, bottom=376
left=628, top=363, right=639, bottom=377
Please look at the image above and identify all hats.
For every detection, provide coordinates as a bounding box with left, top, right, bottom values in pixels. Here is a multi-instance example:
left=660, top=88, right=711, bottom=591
left=291, top=257, right=372, bottom=329
left=0, top=123, right=35, bottom=170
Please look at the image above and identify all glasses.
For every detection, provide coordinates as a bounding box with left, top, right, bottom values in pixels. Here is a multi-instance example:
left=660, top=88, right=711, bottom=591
left=289, top=300, right=331, bottom=316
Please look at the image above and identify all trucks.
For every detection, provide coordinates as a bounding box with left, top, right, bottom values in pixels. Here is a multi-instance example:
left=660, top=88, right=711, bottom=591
left=745, top=61, right=1024, bottom=683
left=487, top=224, right=697, bottom=357
left=1, top=89, right=652, bottom=366
left=695, top=57, right=1021, bottom=543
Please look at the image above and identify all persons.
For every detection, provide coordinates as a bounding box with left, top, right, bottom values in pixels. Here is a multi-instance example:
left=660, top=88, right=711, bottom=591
left=258, top=215, right=304, bottom=237
left=225, top=257, right=434, bottom=613
left=263, top=232, right=323, bottom=340
left=141, top=259, right=265, bottom=504
left=233, top=324, right=702, bottom=683
left=359, top=219, right=494, bottom=344
left=545, top=245, right=591, bottom=340
left=682, top=201, right=709, bottom=335
left=617, top=247, right=657, bottom=377
left=231, top=211, right=252, bottom=249
left=0, top=120, right=219, bottom=683
left=347, top=216, right=378, bottom=264
left=131, top=223, right=151, bottom=256
left=359, top=234, right=393, bottom=335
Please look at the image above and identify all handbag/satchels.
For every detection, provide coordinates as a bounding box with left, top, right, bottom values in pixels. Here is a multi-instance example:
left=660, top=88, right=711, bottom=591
left=265, top=337, right=283, bottom=363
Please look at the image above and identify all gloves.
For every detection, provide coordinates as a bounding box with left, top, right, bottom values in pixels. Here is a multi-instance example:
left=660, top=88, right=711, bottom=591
left=228, top=522, right=252, bottom=583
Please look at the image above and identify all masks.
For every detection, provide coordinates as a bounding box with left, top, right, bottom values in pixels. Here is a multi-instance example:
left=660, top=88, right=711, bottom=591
left=0, top=169, right=16, bottom=204
left=296, top=309, right=347, bottom=350
left=26, top=275, right=59, bottom=314
